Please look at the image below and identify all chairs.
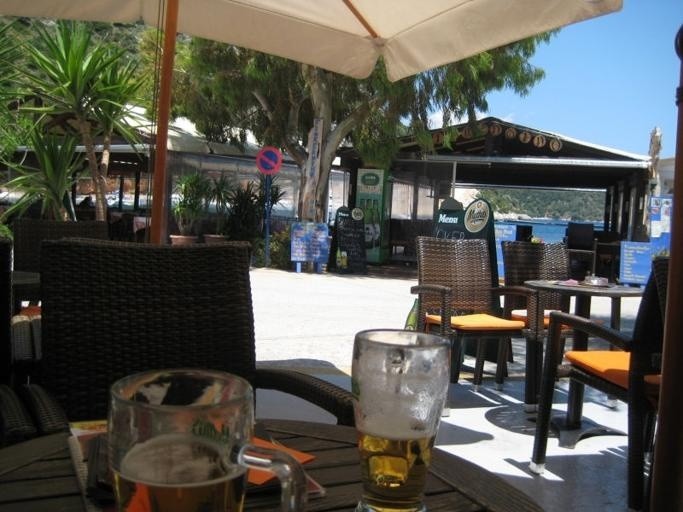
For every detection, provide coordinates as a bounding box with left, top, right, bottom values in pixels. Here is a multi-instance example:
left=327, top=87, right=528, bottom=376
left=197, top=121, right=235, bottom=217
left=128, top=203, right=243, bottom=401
left=13, top=218, right=109, bottom=312
left=24, top=237, right=357, bottom=427
left=564, top=222, right=599, bottom=277
left=1, top=238, right=36, bottom=446
left=528, top=255, right=669, bottom=511
left=411, top=234, right=606, bottom=396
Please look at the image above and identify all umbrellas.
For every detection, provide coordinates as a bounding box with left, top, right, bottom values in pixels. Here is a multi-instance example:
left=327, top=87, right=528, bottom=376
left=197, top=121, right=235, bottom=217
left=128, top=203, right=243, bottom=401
left=0, top=0, right=624, bottom=245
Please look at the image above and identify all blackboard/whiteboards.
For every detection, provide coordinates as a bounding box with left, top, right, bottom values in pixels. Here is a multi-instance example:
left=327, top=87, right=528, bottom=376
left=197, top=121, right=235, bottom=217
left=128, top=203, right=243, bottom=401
left=334, top=208, right=366, bottom=274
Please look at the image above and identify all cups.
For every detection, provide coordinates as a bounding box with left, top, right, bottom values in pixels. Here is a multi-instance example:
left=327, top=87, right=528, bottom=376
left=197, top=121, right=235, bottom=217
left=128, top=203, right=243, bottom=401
left=351, top=330, right=452, bottom=512
left=105, top=367, right=308, bottom=511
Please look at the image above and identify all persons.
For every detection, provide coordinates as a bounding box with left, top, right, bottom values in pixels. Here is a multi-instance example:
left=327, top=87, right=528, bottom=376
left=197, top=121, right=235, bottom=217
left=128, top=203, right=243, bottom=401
left=78, top=196, right=90, bottom=208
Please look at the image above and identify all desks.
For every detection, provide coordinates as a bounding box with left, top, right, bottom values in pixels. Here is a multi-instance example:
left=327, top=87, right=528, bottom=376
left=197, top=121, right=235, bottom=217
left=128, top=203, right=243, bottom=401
left=522, top=278, right=644, bottom=450
left=11, top=269, right=39, bottom=312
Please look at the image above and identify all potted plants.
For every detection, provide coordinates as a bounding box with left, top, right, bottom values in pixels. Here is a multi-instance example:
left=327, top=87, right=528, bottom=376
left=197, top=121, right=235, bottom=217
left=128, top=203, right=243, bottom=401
left=168, top=170, right=287, bottom=271
